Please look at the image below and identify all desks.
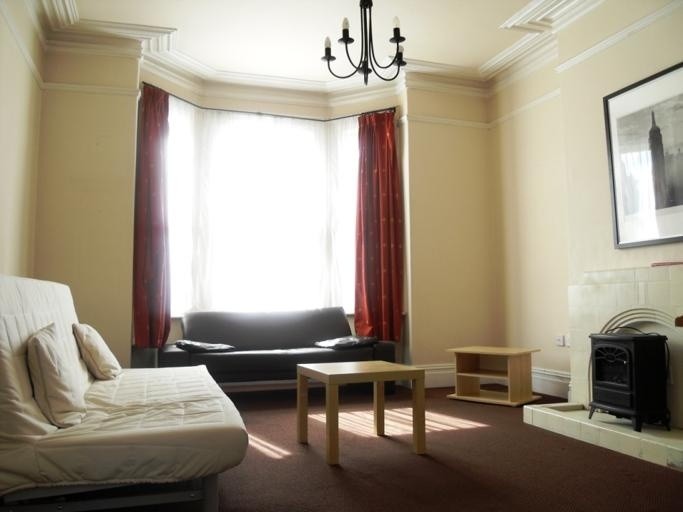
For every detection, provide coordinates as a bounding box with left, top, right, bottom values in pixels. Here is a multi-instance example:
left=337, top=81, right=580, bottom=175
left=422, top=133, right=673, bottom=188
left=292, top=357, right=427, bottom=469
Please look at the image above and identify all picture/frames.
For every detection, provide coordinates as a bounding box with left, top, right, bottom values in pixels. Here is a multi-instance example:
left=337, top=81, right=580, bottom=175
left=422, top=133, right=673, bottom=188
left=600, top=62, right=683, bottom=255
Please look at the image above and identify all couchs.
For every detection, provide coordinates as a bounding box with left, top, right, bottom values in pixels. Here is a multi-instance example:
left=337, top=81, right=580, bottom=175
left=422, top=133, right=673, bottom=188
left=0, top=272, right=251, bottom=512
left=156, top=304, right=399, bottom=396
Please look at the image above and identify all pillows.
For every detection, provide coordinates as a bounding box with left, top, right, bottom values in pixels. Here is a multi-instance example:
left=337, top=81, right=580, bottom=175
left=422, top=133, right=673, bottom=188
left=69, top=319, right=125, bottom=381
left=311, top=330, right=379, bottom=352
left=21, top=319, right=91, bottom=429
left=171, top=336, right=237, bottom=355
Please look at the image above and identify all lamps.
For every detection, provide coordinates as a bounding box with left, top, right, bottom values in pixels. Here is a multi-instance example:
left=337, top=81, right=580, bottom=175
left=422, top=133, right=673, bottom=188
left=318, top=1, right=411, bottom=85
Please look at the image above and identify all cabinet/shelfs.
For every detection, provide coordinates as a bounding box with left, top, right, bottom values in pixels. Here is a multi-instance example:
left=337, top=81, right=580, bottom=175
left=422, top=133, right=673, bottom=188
left=584, top=329, right=673, bottom=435
left=444, top=339, right=543, bottom=410
